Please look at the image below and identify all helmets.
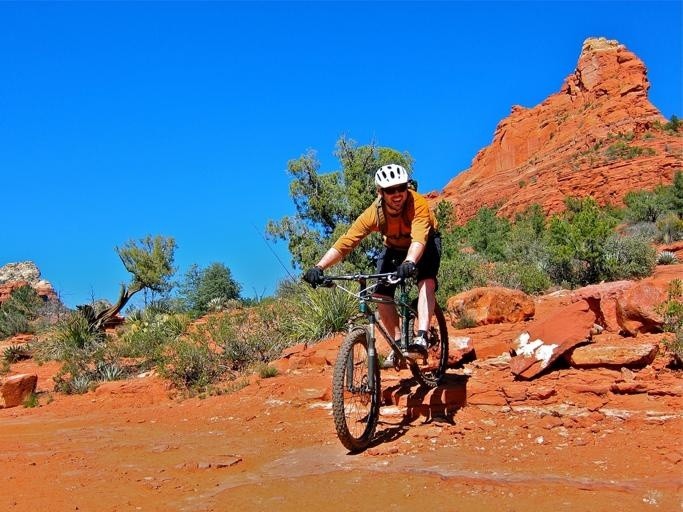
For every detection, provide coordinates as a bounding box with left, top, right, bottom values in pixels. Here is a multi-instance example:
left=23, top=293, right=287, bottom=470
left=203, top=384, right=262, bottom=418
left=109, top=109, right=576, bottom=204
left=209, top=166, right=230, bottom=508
left=374, top=163, right=409, bottom=190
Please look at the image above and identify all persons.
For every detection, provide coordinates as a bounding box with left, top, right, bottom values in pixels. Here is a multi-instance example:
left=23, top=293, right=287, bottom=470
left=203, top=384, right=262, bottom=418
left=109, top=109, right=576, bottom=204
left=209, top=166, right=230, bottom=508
left=304, top=164, right=442, bottom=369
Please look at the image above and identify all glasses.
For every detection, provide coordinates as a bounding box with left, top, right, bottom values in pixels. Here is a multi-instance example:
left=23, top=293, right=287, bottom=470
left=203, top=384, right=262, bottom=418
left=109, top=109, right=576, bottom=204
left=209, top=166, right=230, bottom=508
left=383, top=182, right=408, bottom=195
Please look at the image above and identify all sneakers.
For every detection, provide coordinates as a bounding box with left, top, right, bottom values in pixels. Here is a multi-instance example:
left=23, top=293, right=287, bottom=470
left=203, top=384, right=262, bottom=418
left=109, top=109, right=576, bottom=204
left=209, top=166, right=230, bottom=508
left=403, top=333, right=429, bottom=359
left=383, top=349, right=408, bottom=369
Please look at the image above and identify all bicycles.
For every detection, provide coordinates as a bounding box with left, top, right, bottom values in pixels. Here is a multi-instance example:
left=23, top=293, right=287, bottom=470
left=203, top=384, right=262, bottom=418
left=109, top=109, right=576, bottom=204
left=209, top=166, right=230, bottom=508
left=309, top=267, right=449, bottom=452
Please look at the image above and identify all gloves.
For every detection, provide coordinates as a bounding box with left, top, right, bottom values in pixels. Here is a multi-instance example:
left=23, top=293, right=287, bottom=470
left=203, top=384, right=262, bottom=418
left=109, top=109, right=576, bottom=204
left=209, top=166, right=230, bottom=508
left=304, top=266, right=324, bottom=290
left=396, top=260, right=417, bottom=279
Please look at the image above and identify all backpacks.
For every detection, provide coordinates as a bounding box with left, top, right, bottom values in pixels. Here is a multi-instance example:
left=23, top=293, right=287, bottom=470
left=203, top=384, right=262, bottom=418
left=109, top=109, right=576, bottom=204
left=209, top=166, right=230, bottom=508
left=376, top=179, right=418, bottom=237
